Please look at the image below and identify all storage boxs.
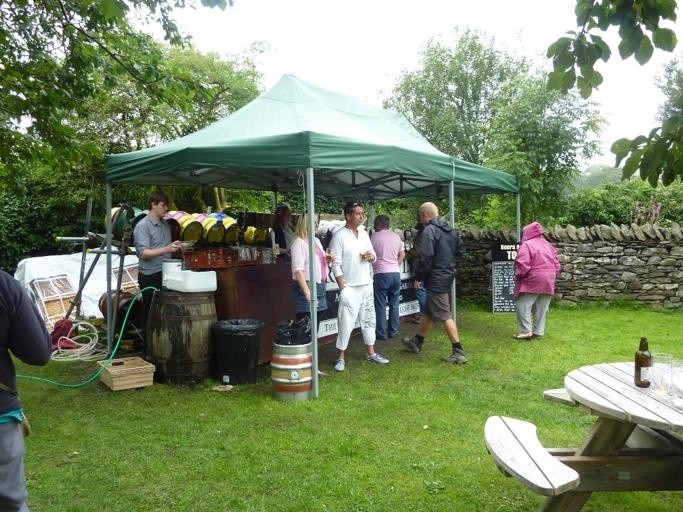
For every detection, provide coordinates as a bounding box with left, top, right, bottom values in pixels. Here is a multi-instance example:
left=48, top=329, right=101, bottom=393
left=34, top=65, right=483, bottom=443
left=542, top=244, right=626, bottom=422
left=97, top=356, right=156, bottom=392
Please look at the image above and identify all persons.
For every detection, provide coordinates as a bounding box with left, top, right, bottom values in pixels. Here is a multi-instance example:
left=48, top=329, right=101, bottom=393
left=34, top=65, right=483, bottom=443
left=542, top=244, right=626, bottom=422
left=0, top=269, right=52, bottom=512
left=401, top=224, right=428, bottom=325
left=513, top=221, right=561, bottom=340
left=268, top=204, right=295, bottom=259
left=370, top=215, right=406, bottom=339
left=133, top=189, right=182, bottom=348
left=291, top=211, right=333, bottom=378
left=401, top=202, right=468, bottom=364
left=328, top=200, right=390, bottom=371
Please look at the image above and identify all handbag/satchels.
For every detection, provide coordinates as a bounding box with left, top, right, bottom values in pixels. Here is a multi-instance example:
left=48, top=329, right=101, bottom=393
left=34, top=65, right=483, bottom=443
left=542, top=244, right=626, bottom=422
left=275, top=316, right=311, bottom=345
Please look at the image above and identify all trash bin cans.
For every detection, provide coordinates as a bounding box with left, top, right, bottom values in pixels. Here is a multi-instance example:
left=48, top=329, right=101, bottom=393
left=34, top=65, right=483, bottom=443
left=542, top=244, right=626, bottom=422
left=210, top=318, right=264, bottom=386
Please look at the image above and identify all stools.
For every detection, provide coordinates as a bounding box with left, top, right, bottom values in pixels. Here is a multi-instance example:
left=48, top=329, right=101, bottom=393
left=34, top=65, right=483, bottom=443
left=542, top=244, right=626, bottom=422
left=483, top=415, right=582, bottom=496
left=543, top=387, right=579, bottom=406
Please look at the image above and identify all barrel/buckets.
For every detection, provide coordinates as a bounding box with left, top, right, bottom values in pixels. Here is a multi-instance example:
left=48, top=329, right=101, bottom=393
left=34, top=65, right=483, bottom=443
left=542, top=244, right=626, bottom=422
left=145, top=290, right=218, bottom=385
left=98, top=290, right=143, bottom=331
left=270, top=341, right=313, bottom=402
left=104, top=206, right=272, bottom=246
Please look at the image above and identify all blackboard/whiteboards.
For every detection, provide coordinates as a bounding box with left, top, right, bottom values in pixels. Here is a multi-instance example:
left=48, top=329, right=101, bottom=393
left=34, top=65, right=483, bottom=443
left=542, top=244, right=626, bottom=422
left=491, top=260, right=520, bottom=314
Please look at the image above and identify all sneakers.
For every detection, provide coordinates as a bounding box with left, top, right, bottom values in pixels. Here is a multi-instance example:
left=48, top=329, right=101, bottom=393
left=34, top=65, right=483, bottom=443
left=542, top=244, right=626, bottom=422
left=402, top=338, right=422, bottom=353
left=412, top=316, right=423, bottom=323
left=513, top=333, right=532, bottom=338
left=444, top=351, right=468, bottom=363
left=534, top=332, right=542, bottom=339
left=334, top=359, right=345, bottom=371
left=367, top=352, right=389, bottom=363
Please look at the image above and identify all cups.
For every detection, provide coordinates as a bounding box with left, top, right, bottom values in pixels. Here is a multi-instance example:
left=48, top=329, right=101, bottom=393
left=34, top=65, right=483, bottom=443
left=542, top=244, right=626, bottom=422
left=650, top=353, right=672, bottom=391
left=326, top=249, right=337, bottom=263
left=359, top=248, right=368, bottom=263
left=670, top=369, right=683, bottom=408
left=236, top=245, right=279, bottom=265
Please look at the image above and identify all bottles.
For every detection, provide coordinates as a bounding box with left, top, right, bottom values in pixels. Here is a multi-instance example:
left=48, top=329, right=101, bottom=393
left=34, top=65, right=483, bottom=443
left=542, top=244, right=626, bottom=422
left=633, top=337, right=652, bottom=387
left=207, top=250, right=216, bottom=266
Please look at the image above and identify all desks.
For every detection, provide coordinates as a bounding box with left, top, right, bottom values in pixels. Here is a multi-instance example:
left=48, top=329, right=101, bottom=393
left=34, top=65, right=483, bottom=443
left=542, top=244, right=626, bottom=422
left=538, top=361, right=683, bottom=512
